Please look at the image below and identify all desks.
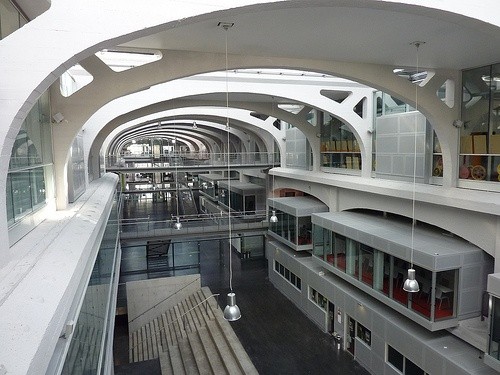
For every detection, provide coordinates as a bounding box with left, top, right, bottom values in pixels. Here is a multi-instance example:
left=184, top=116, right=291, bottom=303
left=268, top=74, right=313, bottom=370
left=361, top=254, right=454, bottom=298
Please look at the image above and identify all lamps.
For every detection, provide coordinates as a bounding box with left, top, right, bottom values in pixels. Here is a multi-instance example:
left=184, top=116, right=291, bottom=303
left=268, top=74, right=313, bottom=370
left=402, top=40, right=427, bottom=293
left=174, top=20, right=279, bottom=321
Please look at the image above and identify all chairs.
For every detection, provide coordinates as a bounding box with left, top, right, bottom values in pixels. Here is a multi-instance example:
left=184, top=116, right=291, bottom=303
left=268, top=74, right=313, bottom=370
left=363, top=258, right=451, bottom=311
